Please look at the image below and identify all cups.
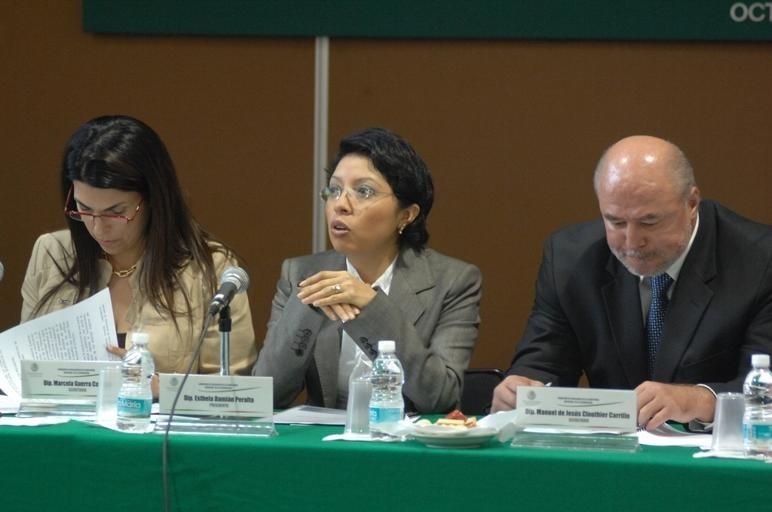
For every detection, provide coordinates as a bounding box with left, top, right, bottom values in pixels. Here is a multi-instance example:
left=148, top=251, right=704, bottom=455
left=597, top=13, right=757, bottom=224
left=345, top=377, right=370, bottom=438
left=96, top=368, right=121, bottom=432
left=707, top=392, right=751, bottom=456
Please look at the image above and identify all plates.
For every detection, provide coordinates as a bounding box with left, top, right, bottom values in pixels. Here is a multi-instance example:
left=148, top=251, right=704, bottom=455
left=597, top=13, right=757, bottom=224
left=410, top=426, right=502, bottom=449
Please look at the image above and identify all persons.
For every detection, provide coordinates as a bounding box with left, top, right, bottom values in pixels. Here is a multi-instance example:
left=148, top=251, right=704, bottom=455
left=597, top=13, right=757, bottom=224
left=489, top=134, right=772, bottom=431
left=18, top=115, right=259, bottom=402
left=251, top=127, right=483, bottom=414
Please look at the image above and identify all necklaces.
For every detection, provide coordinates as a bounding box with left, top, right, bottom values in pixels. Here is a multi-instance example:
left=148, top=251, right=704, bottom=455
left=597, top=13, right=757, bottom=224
left=104, top=253, right=137, bottom=278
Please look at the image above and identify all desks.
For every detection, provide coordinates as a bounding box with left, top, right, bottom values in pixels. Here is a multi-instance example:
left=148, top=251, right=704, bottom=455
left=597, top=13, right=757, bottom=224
left=0, top=393, right=771, bottom=512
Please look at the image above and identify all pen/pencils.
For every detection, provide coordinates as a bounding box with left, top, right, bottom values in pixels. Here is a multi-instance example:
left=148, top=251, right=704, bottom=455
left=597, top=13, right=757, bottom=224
left=544, top=381, right=554, bottom=388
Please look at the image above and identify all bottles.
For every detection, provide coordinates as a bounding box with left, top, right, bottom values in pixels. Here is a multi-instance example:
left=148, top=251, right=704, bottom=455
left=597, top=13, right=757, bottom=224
left=116, top=333, right=155, bottom=432
left=742, top=352, right=772, bottom=463
left=367, top=339, right=406, bottom=442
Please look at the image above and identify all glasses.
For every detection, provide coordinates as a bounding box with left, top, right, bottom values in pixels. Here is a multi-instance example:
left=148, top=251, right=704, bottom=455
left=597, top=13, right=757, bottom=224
left=319, top=183, right=396, bottom=203
left=62, top=183, right=149, bottom=227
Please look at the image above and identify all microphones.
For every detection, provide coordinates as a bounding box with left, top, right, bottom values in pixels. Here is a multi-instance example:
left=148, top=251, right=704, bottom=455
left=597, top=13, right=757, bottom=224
left=209, top=266, right=250, bottom=315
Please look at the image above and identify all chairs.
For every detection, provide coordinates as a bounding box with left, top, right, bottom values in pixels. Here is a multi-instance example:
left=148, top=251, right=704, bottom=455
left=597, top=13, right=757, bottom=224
left=457, top=367, right=507, bottom=415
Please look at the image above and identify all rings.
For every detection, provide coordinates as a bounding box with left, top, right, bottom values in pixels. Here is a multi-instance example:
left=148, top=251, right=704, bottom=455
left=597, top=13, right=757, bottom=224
left=335, top=284, right=341, bottom=293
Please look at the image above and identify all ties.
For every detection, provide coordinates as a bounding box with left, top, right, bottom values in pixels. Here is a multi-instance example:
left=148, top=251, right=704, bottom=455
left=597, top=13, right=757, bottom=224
left=644, top=274, right=672, bottom=377
